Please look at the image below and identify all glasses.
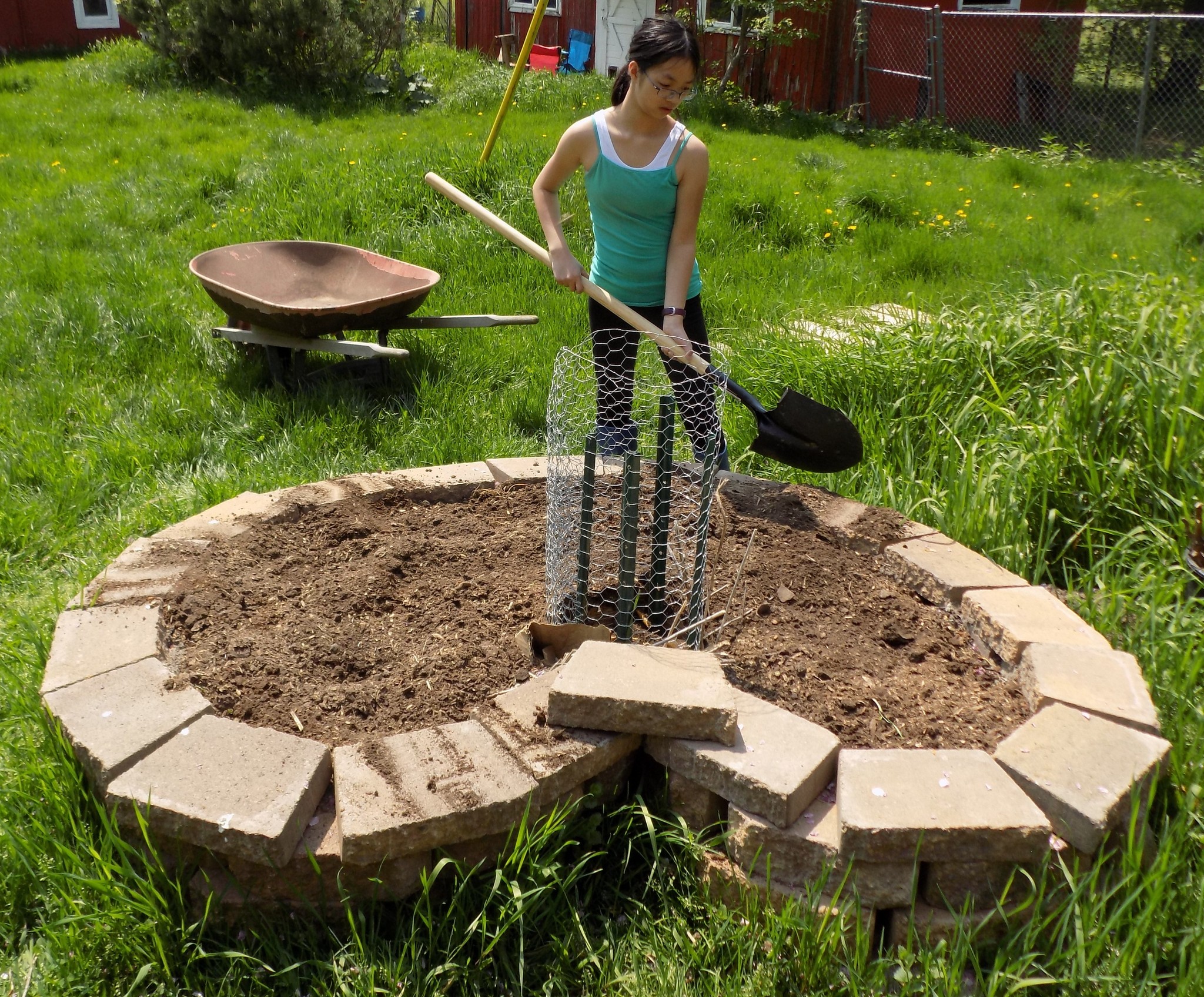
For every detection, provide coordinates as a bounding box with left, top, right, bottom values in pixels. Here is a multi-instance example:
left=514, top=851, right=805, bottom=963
left=643, top=70, right=698, bottom=101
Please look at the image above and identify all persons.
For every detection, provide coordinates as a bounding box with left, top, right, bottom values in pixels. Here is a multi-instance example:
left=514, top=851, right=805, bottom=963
left=533, top=14, right=730, bottom=472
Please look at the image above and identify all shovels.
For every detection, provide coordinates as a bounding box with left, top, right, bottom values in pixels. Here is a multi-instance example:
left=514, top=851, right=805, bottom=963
left=424, top=172, right=863, bottom=473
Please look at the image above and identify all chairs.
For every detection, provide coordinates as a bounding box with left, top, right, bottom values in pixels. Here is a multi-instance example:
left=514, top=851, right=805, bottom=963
left=501, top=35, right=529, bottom=72
left=524, top=28, right=593, bottom=77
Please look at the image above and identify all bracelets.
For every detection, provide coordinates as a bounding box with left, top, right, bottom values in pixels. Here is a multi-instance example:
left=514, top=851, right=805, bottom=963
left=661, top=307, right=687, bottom=319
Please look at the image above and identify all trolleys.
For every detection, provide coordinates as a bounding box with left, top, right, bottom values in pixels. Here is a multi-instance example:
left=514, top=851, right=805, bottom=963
left=189, top=239, right=542, bottom=410
